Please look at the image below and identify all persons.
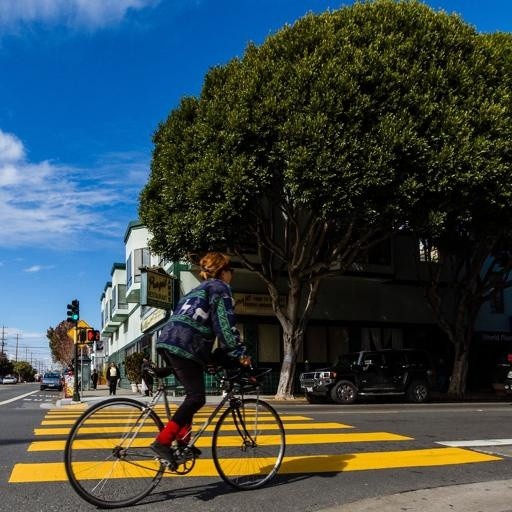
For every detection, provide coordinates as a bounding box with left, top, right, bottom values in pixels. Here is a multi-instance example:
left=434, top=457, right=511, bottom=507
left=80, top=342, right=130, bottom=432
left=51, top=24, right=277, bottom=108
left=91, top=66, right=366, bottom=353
left=142, top=356, right=154, bottom=396
left=91, top=369, right=98, bottom=390
left=152, top=249, right=252, bottom=471
left=106, top=361, right=121, bottom=395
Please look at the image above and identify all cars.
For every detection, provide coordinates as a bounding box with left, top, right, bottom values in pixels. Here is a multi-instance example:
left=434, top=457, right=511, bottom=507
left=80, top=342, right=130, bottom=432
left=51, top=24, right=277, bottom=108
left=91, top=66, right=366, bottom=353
left=3, top=375, right=18, bottom=385
left=40, top=372, right=65, bottom=391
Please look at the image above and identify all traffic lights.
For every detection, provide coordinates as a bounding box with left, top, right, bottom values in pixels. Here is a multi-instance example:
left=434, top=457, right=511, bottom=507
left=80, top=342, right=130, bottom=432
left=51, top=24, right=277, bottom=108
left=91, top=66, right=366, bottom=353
left=86, top=329, right=101, bottom=342
left=67, top=299, right=80, bottom=324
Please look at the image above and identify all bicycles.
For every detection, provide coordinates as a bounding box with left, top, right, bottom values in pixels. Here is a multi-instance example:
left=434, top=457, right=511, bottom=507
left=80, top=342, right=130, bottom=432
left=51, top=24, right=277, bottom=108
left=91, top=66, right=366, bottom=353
left=64, top=365, right=286, bottom=509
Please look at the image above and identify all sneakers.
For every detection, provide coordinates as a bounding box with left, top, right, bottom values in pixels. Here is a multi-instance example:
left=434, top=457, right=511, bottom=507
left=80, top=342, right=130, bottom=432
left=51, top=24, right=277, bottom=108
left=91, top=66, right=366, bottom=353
left=149, top=439, right=179, bottom=471
left=177, top=444, right=202, bottom=459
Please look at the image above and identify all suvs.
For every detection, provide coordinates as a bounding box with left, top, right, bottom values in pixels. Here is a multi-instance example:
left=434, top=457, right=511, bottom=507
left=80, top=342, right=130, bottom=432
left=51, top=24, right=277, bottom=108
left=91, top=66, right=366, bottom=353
left=298, top=347, right=437, bottom=405
